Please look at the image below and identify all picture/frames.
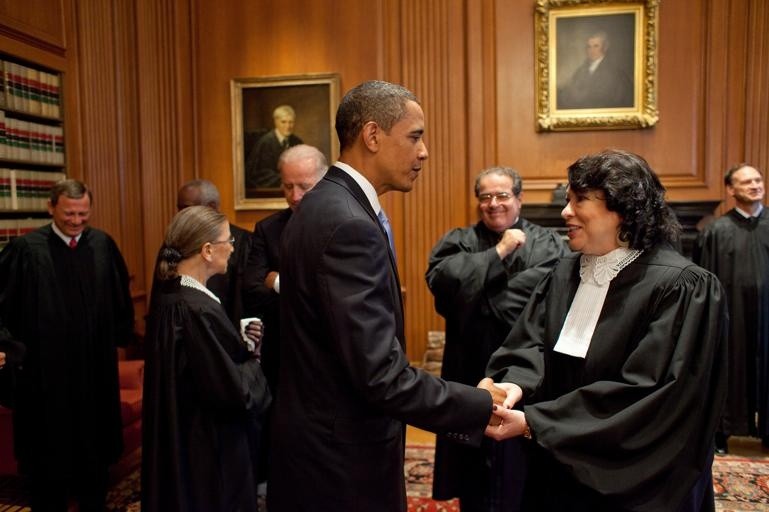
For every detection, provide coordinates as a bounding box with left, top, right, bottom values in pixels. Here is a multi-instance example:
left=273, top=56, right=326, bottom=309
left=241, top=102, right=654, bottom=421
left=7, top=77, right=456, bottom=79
left=228, top=72, right=341, bottom=213
left=530, top=1, right=661, bottom=135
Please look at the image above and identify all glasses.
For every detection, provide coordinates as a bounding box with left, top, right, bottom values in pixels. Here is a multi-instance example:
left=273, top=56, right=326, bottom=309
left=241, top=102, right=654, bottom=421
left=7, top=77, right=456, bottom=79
left=479, top=193, right=512, bottom=202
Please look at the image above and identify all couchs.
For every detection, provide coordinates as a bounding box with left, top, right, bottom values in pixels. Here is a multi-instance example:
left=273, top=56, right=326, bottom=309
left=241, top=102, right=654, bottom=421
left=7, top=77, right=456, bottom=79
left=0, top=358, right=146, bottom=490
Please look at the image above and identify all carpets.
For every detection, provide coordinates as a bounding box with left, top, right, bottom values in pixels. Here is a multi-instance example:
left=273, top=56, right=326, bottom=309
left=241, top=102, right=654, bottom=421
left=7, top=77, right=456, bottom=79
left=0, top=441, right=768, bottom=511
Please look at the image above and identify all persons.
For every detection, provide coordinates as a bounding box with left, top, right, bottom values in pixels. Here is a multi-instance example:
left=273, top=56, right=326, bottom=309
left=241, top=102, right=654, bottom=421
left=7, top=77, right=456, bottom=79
left=265, top=81, right=507, bottom=512
left=140, top=144, right=330, bottom=512
left=425, top=167, right=584, bottom=512
left=0, top=175, right=135, bottom=509
left=561, top=23, right=624, bottom=108
left=694, top=159, right=768, bottom=459
left=484, top=147, right=726, bottom=512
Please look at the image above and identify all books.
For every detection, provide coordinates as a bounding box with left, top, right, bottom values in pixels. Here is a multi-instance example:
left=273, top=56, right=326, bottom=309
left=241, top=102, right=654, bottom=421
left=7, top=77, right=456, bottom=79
left=0, top=56, right=71, bottom=250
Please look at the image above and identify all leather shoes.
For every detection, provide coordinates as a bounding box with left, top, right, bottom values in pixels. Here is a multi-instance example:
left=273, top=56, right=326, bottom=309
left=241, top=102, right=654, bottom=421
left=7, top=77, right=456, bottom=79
left=715, top=424, right=728, bottom=456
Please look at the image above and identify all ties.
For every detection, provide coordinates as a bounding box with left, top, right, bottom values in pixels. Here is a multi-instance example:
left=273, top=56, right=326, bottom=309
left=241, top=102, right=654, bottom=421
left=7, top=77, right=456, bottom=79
left=70, top=239, right=77, bottom=249
left=379, top=209, right=395, bottom=262
